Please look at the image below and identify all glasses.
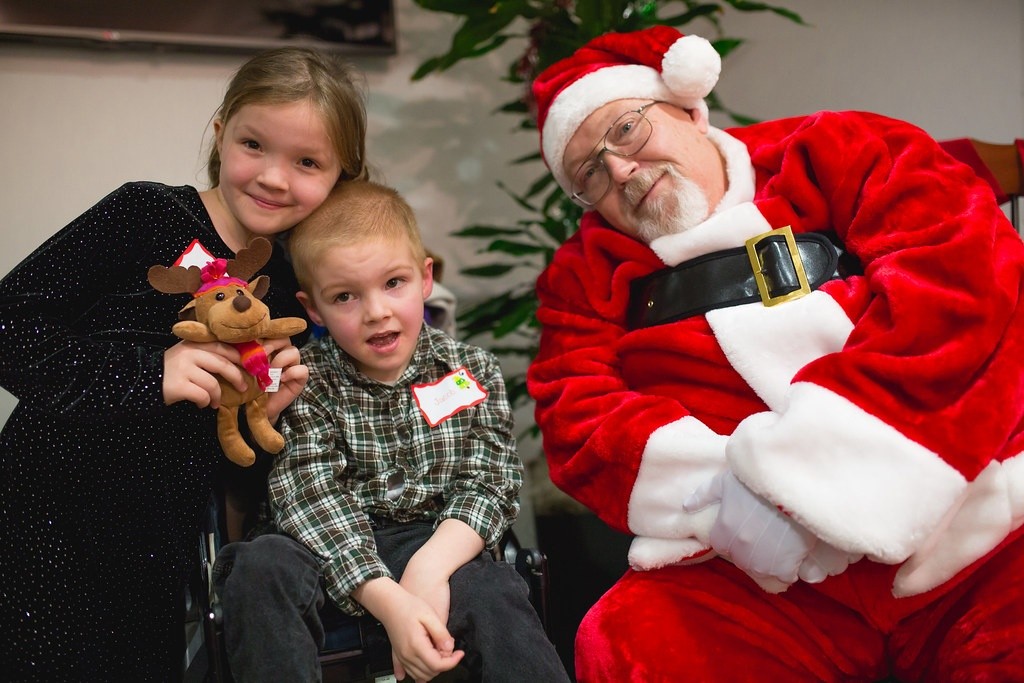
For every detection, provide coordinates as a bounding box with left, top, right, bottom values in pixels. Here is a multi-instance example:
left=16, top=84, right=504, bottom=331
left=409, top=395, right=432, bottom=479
left=570, top=100, right=665, bottom=206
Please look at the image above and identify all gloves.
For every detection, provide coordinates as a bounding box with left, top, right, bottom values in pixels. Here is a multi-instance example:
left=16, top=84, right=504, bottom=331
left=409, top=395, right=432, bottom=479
left=682, top=465, right=818, bottom=584
left=799, top=539, right=865, bottom=583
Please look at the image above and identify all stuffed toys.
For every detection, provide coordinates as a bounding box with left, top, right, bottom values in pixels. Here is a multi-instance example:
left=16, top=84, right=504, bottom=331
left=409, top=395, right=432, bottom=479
left=147, top=236, right=308, bottom=466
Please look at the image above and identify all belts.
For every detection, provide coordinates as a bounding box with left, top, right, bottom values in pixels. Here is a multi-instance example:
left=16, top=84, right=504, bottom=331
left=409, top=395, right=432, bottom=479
left=626, top=226, right=863, bottom=332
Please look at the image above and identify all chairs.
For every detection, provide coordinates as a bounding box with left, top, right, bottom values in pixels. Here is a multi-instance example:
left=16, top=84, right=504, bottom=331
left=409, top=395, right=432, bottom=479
left=195, top=449, right=549, bottom=683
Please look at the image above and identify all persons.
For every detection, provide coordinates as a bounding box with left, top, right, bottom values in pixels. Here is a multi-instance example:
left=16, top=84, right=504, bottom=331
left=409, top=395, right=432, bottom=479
left=526, top=24, right=1024, bottom=683
left=0, top=49, right=370, bottom=682
left=211, top=178, right=575, bottom=682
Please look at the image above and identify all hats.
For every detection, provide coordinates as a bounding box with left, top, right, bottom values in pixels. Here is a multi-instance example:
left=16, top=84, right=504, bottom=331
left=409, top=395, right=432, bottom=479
left=530, top=25, right=721, bottom=213
